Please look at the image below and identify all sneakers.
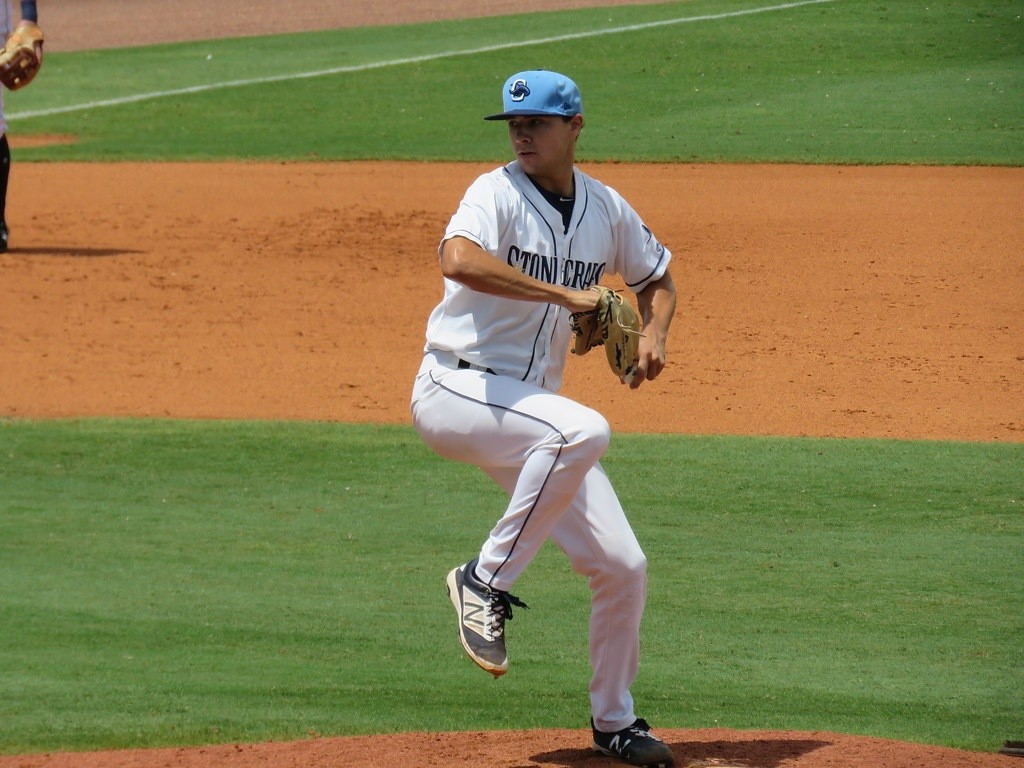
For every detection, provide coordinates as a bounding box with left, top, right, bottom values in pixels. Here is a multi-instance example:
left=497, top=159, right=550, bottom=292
left=447, top=557, right=530, bottom=680
left=590, top=715, right=675, bottom=768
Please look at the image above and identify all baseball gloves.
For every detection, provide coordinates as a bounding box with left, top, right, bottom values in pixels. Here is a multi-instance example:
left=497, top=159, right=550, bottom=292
left=0, top=22, right=44, bottom=91
left=571, top=286, right=644, bottom=386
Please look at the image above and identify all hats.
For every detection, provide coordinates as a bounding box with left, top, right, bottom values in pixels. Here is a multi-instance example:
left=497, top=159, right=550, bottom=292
left=484, top=69, right=582, bottom=120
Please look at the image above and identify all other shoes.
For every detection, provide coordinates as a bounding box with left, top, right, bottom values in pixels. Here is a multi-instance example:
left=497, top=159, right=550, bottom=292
left=0, top=229, right=7, bottom=252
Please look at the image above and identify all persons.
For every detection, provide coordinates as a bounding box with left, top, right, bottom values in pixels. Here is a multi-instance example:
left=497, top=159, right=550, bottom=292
left=407, top=69, right=680, bottom=766
left=0, top=0, right=45, bottom=251
